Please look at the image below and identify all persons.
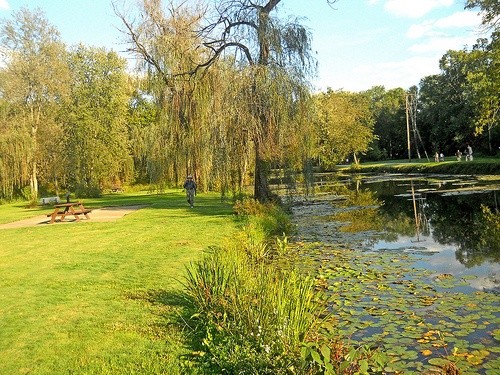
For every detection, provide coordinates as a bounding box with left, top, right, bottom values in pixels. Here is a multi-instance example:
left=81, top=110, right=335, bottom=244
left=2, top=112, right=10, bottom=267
left=433, top=152, right=439, bottom=163
left=184, top=176, right=196, bottom=209
left=439, top=153, right=444, bottom=161
left=465, top=145, right=473, bottom=160
left=455, top=150, right=462, bottom=161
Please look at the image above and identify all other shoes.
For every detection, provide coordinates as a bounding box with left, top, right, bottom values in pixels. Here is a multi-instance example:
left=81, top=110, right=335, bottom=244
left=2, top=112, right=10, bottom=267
left=190, top=205, right=194, bottom=209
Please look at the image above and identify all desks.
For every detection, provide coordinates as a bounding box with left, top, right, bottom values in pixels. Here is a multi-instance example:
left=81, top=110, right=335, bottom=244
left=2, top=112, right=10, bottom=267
left=47, top=202, right=92, bottom=224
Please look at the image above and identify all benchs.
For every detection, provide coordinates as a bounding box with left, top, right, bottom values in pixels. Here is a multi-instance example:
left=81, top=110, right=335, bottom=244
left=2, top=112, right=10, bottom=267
left=74, top=208, right=91, bottom=215
left=50, top=210, right=64, bottom=215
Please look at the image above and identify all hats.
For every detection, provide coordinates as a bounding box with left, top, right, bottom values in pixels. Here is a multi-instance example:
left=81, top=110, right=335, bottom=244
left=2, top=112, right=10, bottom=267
left=187, top=176, right=193, bottom=179
